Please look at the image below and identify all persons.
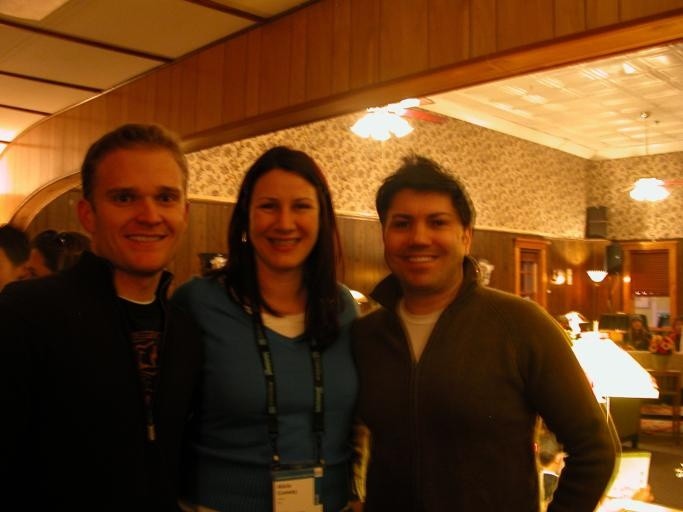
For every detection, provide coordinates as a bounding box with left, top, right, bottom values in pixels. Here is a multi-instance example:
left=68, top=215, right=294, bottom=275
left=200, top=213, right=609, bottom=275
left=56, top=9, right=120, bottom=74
left=623, top=312, right=655, bottom=352
left=0, top=121, right=191, bottom=512
left=152, top=144, right=362, bottom=512
left=646, top=312, right=683, bottom=354
left=322, top=157, right=617, bottom=512
left=1, top=224, right=92, bottom=290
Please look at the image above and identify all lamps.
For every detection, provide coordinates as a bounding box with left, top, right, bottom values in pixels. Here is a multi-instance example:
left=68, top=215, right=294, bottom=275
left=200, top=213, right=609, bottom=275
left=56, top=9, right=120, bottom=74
left=346, top=95, right=424, bottom=141
left=586, top=269, right=608, bottom=283
left=628, top=111, right=671, bottom=202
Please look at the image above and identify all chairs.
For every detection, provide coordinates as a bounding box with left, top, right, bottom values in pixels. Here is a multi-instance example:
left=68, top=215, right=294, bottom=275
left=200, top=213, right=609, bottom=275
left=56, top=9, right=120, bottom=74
left=643, top=367, right=681, bottom=442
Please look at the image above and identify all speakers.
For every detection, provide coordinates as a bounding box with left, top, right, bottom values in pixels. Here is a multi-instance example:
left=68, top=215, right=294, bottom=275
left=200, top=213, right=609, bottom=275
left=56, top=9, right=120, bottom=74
left=607, top=245, right=623, bottom=274
left=586, top=207, right=608, bottom=238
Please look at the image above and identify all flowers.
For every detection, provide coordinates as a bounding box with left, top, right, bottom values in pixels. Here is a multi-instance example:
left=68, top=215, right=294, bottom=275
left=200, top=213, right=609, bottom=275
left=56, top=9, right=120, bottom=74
left=646, top=332, right=677, bottom=357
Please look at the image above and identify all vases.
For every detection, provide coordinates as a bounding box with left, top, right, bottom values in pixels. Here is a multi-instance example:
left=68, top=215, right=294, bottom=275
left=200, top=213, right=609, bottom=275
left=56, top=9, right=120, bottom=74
left=649, top=353, right=669, bottom=370
